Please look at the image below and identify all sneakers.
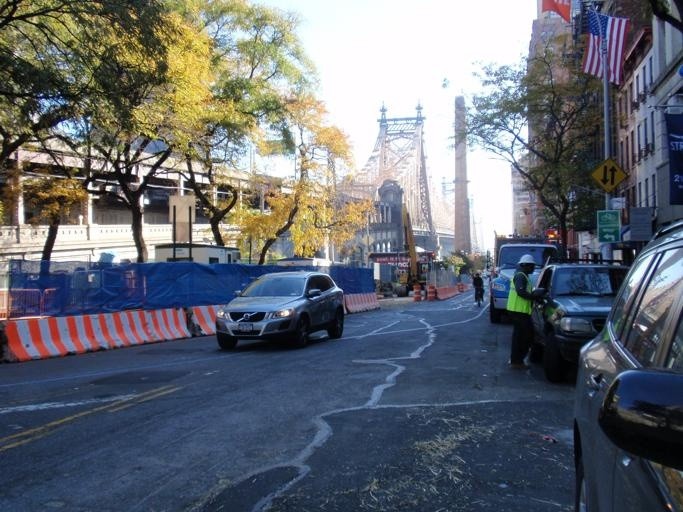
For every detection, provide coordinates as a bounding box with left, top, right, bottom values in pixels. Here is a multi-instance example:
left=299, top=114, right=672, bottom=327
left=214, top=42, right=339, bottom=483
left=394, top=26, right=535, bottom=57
left=511, top=363, right=530, bottom=370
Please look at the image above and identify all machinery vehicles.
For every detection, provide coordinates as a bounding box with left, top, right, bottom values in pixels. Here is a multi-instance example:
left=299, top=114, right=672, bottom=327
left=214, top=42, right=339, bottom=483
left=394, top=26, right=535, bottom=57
left=396, top=206, right=425, bottom=296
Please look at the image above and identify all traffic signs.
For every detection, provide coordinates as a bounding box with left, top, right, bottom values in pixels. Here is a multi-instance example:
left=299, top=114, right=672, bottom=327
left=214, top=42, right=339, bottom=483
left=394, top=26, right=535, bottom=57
left=596, top=209, right=622, bottom=243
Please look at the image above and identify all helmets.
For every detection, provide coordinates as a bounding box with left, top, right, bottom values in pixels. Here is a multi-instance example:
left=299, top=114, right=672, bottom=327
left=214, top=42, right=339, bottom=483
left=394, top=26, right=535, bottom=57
left=517, top=254, right=537, bottom=266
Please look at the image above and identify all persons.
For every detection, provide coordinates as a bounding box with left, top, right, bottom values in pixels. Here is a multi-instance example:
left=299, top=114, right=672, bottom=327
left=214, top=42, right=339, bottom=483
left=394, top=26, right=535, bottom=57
left=472, top=273, right=485, bottom=302
left=506, top=253, right=544, bottom=371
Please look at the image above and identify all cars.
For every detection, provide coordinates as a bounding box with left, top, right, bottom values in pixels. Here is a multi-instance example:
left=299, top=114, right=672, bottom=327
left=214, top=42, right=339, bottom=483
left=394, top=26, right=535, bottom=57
left=215, top=270, right=344, bottom=349
left=530, top=261, right=630, bottom=383
left=571, top=220, right=683, bottom=511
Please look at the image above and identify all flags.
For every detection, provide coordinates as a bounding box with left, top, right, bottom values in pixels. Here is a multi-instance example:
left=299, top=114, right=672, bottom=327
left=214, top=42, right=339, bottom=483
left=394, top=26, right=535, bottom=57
left=542, top=0, right=572, bottom=24
left=581, top=9, right=630, bottom=87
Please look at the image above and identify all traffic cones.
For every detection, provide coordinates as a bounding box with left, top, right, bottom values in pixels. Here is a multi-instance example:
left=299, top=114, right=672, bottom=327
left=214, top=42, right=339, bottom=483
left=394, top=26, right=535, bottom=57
left=412, top=283, right=421, bottom=300
left=426, top=283, right=435, bottom=301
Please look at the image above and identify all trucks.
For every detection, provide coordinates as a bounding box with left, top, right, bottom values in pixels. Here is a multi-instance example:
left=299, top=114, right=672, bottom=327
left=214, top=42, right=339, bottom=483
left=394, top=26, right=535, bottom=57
left=489, top=235, right=562, bottom=324
